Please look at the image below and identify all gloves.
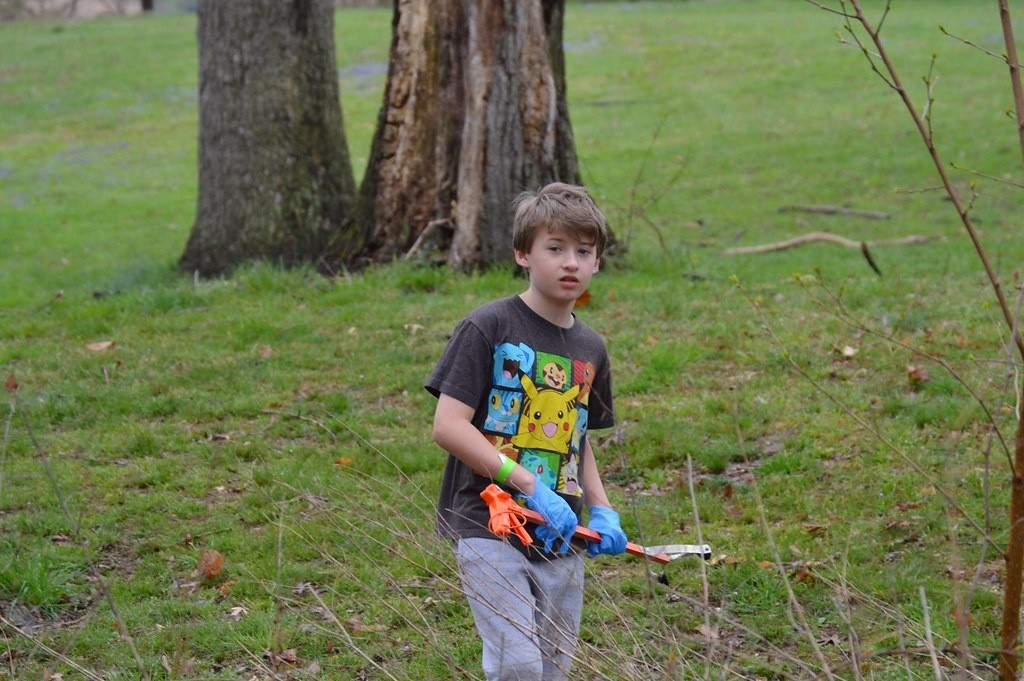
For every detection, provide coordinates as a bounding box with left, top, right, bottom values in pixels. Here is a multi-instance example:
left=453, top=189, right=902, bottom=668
left=585, top=505, right=628, bottom=558
left=519, top=473, right=579, bottom=554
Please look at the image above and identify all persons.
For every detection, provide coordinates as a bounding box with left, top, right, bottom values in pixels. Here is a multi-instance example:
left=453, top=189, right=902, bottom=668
left=425, top=181, right=626, bottom=681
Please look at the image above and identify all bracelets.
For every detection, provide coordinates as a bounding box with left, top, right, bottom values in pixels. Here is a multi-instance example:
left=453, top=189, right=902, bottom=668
left=494, top=454, right=516, bottom=484
